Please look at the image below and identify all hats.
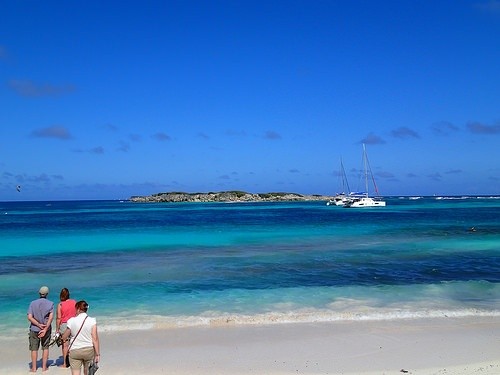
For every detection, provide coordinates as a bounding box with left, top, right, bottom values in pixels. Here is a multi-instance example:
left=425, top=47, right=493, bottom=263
left=39, top=286, right=49, bottom=294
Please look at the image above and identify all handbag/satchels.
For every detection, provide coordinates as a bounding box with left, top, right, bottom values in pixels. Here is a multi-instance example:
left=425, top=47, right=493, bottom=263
left=66, top=355, right=70, bottom=367
left=55, top=333, right=63, bottom=347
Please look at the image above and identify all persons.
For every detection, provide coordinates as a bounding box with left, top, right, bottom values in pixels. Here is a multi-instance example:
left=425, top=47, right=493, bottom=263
left=56, top=288, right=78, bottom=368
left=63, top=300, right=100, bottom=375
left=27, top=286, right=53, bottom=372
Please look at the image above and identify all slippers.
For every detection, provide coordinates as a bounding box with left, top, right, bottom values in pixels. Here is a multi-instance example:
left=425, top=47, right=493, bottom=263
left=93, top=359, right=98, bottom=375
left=89, top=360, right=93, bottom=375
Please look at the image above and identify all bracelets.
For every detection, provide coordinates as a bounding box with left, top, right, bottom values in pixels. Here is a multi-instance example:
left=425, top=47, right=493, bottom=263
left=96, top=354, right=100, bottom=356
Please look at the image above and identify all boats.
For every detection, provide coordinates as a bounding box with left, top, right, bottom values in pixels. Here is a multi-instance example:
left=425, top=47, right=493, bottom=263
left=346, top=142, right=385, bottom=208
left=326, top=156, right=362, bottom=207
